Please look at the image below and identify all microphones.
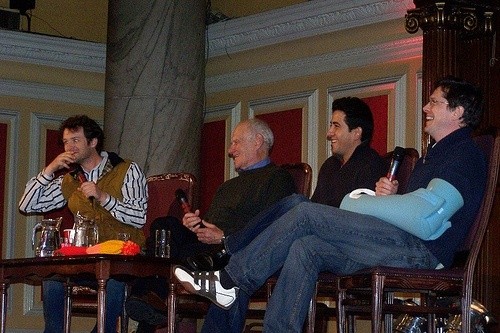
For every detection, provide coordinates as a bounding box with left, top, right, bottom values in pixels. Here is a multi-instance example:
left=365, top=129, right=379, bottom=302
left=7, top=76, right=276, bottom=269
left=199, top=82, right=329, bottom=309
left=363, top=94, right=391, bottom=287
left=175, top=188, right=201, bottom=229
left=75, top=166, right=95, bottom=201
left=387, top=145, right=406, bottom=182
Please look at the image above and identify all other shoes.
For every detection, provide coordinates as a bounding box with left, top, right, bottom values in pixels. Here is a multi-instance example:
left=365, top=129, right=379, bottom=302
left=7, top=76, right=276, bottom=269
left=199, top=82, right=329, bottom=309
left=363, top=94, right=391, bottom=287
left=125, top=290, right=168, bottom=327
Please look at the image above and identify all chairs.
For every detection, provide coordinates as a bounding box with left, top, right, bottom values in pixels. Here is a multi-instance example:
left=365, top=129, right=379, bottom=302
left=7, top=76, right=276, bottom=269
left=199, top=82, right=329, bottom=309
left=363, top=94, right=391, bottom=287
left=71, top=172, right=193, bottom=315
left=335, top=134, right=500, bottom=333
left=264, top=148, right=419, bottom=333
left=121, top=162, right=311, bottom=333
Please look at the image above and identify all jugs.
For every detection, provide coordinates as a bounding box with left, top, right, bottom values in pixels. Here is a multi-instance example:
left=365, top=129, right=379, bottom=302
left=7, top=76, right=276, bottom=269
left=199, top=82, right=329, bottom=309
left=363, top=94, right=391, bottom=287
left=74, top=215, right=99, bottom=247
left=32, top=216, right=63, bottom=257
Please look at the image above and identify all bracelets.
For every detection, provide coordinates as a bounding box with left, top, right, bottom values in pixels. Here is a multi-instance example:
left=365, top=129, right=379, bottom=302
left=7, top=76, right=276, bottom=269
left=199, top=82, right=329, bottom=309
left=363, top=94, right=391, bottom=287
left=220, top=236, right=225, bottom=243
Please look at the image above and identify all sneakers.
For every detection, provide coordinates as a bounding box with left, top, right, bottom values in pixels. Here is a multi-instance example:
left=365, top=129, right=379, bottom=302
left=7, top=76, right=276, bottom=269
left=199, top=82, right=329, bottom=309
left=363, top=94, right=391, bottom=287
left=173, top=265, right=241, bottom=310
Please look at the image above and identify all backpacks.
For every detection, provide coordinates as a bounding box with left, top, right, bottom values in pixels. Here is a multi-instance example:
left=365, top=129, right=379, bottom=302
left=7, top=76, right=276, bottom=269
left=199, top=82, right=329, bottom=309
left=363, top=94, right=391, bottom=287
left=145, top=215, right=222, bottom=274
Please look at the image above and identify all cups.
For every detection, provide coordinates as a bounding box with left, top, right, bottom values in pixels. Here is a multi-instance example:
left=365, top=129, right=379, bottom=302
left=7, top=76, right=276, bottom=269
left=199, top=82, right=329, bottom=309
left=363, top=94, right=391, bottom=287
left=117, top=232, right=130, bottom=241
left=63, top=229, right=75, bottom=243
left=155, top=230, right=170, bottom=258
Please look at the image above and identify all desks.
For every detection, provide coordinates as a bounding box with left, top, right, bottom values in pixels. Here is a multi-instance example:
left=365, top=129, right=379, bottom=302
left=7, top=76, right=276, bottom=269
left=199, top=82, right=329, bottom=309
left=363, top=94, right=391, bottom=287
left=0, top=254, right=192, bottom=333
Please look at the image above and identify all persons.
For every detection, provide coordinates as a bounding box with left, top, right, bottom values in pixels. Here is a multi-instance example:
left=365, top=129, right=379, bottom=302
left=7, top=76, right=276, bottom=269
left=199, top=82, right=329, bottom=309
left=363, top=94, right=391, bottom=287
left=185, top=94, right=390, bottom=333
left=18, top=115, right=149, bottom=333
left=171, top=80, right=488, bottom=333
left=125, top=118, right=296, bottom=333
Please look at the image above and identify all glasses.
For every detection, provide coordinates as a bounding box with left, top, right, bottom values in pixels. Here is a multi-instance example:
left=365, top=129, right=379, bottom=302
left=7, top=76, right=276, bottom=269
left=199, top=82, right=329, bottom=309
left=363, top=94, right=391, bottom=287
left=426, top=99, right=449, bottom=106
